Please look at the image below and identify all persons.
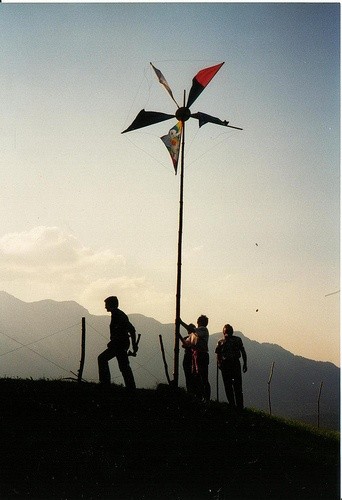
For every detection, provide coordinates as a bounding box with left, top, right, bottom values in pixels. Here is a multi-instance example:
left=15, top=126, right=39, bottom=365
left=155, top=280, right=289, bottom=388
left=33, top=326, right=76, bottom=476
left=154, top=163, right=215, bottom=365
left=183, top=322, right=196, bottom=398
left=180, top=313, right=211, bottom=400
left=97, top=296, right=138, bottom=389
left=216, top=322, right=248, bottom=408
